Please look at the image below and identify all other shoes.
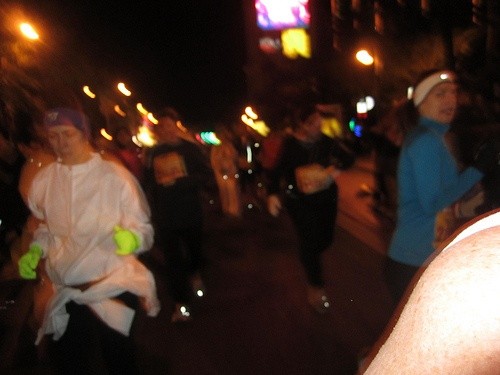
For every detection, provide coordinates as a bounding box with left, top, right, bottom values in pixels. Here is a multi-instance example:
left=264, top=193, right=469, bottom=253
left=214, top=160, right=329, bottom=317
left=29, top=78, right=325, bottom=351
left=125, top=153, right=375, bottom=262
left=306, top=288, right=332, bottom=314
left=188, top=281, right=208, bottom=302
left=171, top=304, right=191, bottom=324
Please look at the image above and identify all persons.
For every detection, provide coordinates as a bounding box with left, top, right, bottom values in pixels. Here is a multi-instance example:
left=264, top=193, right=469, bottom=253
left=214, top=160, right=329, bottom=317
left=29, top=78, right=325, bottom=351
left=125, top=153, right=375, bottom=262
left=11, top=64, right=500, bottom=375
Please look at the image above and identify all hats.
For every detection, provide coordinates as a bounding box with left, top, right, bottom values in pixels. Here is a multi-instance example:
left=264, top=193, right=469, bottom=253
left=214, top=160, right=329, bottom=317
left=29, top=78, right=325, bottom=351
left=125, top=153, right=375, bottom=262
left=412, top=66, right=457, bottom=106
left=43, top=88, right=89, bottom=138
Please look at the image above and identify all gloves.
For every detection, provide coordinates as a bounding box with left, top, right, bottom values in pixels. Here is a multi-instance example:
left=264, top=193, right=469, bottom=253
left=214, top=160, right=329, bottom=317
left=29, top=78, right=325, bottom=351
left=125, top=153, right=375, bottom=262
left=17, top=243, right=43, bottom=281
left=113, top=223, right=140, bottom=256
